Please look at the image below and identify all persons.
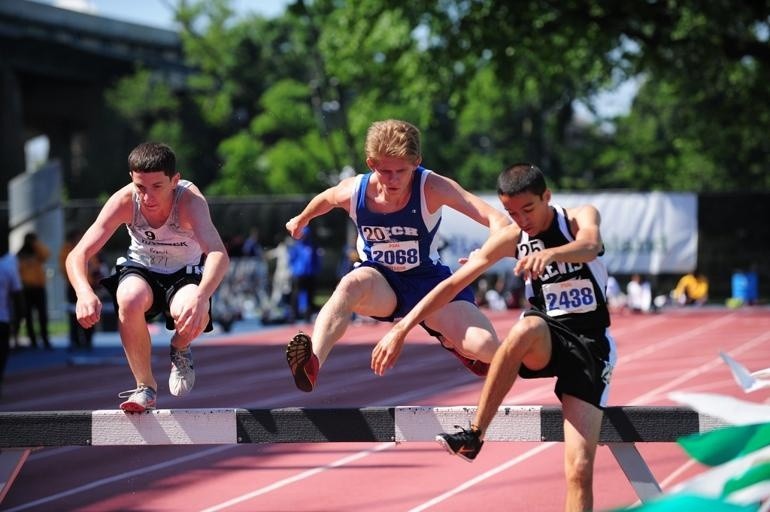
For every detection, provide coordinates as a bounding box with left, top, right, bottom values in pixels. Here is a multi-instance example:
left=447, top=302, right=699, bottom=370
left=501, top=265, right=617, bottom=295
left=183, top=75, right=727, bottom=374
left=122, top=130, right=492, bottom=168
left=226, top=229, right=316, bottom=323
left=62, top=139, right=231, bottom=413
left=1, top=233, right=51, bottom=351
left=61, top=233, right=108, bottom=351
left=284, top=120, right=513, bottom=395
left=472, top=268, right=762, bottom=317
left=368, top=161, right=617, bottom=512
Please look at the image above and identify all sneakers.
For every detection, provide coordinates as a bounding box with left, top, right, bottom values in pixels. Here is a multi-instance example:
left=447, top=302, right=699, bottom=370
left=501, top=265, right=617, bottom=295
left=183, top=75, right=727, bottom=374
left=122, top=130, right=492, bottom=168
left=169, top=339, right=195, bottom=397
left=435, top=425, right=484, bottom=463
left=451, top=349, right=489, bottom=377
left=120, top=383, right=158, bottom=413
left=285, top=333, right=320, bottom=393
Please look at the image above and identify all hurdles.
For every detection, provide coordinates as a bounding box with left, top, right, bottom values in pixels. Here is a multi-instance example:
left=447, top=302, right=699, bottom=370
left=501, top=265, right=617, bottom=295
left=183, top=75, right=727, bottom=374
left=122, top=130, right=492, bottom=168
left=1, top=404, right=699, bottom=512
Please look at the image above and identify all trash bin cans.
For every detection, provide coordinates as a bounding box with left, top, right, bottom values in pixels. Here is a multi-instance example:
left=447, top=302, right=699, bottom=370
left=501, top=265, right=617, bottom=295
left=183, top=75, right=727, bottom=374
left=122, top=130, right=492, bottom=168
left=731, top=271, right=759, bottom=302
